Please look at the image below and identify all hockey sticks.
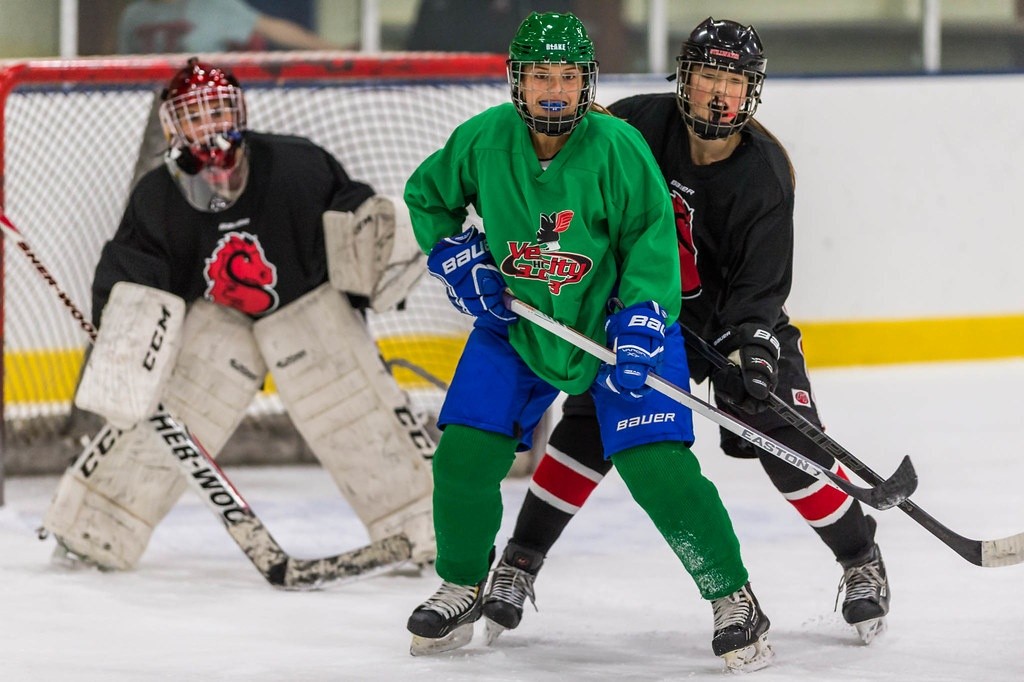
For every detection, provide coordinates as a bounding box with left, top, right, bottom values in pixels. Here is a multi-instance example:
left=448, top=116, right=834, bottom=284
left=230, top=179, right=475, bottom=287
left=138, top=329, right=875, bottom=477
left=679, top=317, right=1022, bottom=570
left=2, top=215, right=413, bottom=592
left=502, top=294, right=920, bottom=511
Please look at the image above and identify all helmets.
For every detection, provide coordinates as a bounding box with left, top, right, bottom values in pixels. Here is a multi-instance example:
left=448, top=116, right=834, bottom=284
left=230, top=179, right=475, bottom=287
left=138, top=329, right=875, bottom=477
left=504, top=10, right=600, bottom=138
left=676, top=16, right=769, bottom=144
left=158, top=57, right=249, bottom=178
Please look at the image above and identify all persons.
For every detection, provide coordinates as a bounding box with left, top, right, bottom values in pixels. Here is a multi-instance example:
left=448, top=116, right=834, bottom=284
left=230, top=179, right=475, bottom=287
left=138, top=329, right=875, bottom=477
left=45, top=60, right=436, bottom=577
left=117, top=0, right=336, bottom=53
left=404, top=8, right=775, bottom=673
left=482, top=16, right=890, bottom=644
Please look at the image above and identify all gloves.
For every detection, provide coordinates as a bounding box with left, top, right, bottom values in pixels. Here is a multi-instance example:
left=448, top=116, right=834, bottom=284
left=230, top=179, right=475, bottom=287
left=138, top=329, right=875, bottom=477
left=424, top=224, right=520, bottom=327
left=587, top=300, right=668, bottom=405
left=713, top=322, right=781, bottom=419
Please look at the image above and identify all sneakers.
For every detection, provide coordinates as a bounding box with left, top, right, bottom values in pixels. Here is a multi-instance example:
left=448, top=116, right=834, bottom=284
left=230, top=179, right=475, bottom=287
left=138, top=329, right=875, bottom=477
left=833, top=514, right=892, bottom=647
left=406, top=544, right=496, bottom=656
left=710, top=581, right=776, bottom=674
left=481, top=537, right=545, bottom=648
